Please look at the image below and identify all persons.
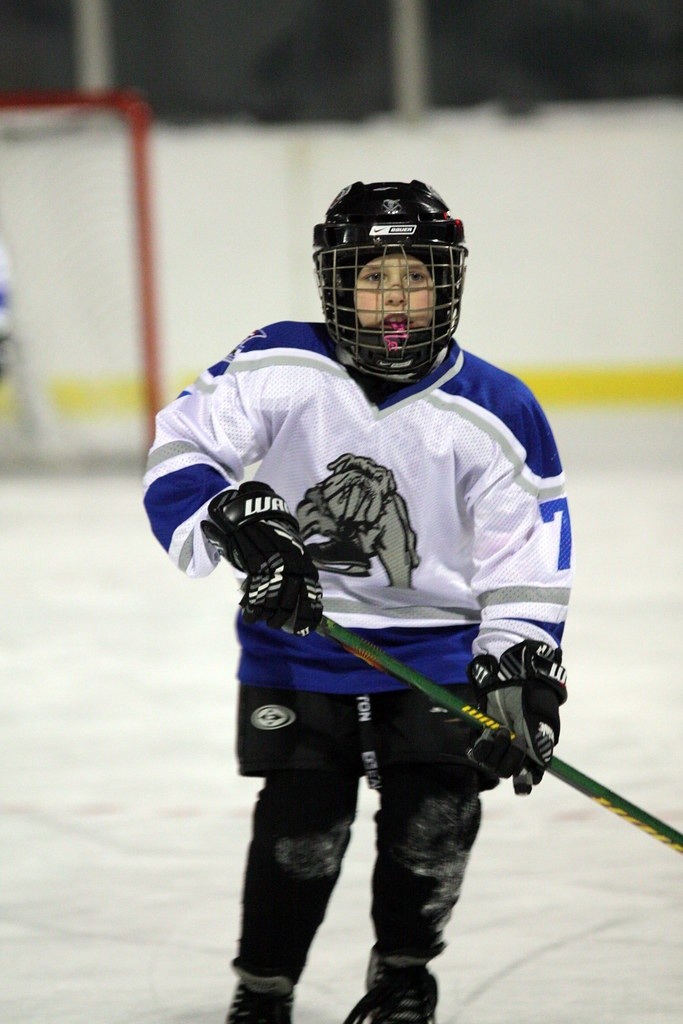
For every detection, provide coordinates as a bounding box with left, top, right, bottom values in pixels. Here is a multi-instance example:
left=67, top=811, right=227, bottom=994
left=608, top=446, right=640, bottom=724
left=144, top=178, right=571, bottom=1024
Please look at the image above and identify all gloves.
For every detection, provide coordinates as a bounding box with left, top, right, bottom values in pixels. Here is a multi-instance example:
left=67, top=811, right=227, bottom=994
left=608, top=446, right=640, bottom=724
left=466, top=640, right=568, bottom=794
left=200, top=480, right=323, bottom=637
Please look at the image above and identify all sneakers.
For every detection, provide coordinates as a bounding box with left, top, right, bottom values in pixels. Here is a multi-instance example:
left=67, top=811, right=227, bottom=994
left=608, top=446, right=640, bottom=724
left=224, top=956, right=294, bottom=1024
left=343, top=940, right=447, bottom=1024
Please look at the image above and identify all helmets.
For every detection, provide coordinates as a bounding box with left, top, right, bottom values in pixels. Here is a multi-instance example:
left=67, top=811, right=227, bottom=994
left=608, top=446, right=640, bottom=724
left=312, top=180, right=468, bottom=382
